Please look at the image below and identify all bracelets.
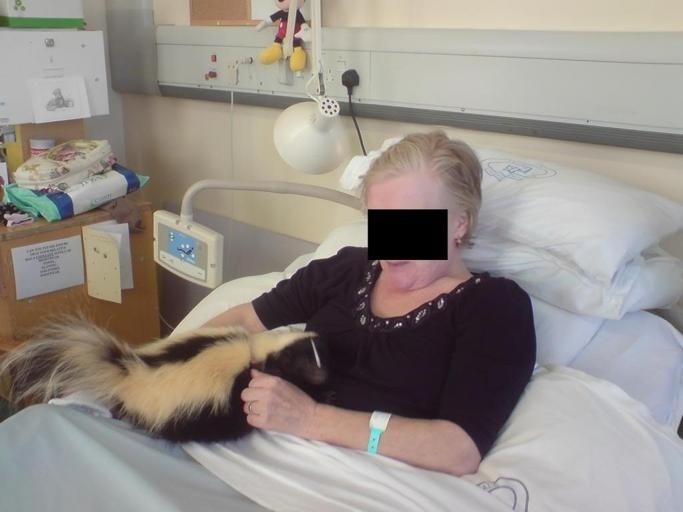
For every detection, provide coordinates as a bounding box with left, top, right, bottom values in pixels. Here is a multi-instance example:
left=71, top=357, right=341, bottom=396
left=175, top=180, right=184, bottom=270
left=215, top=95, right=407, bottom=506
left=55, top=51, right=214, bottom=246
left=363, top=407, right=393, bottom=457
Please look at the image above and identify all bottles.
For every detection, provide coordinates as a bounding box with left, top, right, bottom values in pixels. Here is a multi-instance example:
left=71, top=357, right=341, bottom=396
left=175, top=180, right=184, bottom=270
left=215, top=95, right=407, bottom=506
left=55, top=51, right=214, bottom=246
left=0, top=102, right=15, bottom=144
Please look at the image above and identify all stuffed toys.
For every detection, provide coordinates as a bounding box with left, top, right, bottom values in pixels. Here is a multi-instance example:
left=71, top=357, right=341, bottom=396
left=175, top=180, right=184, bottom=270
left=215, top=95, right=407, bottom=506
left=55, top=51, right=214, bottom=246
left=253, top=0, right=314, bottom=74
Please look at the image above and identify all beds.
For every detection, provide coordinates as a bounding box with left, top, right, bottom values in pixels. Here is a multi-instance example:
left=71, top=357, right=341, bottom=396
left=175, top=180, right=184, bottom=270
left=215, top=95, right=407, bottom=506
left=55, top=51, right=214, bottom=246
left=0, top=220, right=683, bottom=512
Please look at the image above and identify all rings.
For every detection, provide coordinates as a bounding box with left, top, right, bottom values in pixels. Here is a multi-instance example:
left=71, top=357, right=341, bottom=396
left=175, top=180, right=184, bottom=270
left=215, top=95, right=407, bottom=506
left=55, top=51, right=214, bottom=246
left=246, top=400, right=255, bottom=415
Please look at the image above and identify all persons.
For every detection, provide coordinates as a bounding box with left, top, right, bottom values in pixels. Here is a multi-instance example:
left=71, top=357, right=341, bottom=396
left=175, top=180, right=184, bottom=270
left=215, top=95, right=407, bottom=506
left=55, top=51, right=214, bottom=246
left=199, top=131, right=538, bottom=481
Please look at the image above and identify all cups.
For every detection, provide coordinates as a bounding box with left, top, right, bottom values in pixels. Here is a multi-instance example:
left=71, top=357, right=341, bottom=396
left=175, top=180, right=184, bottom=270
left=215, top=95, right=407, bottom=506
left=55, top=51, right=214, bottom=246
left=30, top=139, right=55, bottom=159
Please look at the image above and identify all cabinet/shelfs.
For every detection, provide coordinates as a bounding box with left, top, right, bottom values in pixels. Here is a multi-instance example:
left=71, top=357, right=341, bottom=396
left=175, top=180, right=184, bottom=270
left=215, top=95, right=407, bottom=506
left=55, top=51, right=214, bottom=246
left=0, top=205, right=158, bottom=412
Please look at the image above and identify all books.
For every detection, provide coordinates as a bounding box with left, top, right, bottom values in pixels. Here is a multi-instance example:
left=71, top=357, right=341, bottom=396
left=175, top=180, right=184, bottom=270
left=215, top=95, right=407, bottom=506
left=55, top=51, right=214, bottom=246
left=80, top=219, right=138, bottom=304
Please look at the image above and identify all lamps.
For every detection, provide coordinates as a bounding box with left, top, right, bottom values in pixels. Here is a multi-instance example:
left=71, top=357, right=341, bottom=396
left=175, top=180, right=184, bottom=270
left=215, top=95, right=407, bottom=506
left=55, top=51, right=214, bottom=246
left=271, top=0, right=349, bottom=176
